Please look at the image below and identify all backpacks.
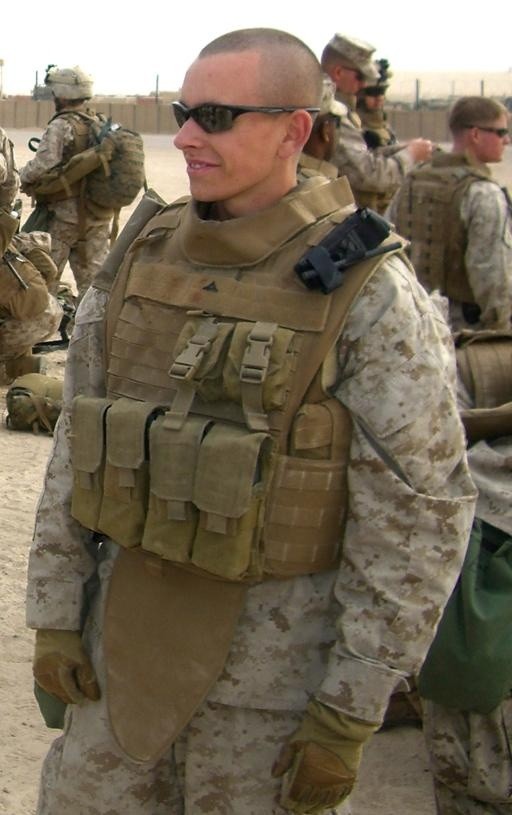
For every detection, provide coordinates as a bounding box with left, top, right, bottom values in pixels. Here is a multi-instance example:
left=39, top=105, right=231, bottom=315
left=62, top=112, right=144, bottom=271
left=0, top=243, right=58, bottom=322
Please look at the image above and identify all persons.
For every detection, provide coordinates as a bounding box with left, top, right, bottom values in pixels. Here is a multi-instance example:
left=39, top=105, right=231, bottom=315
left=320, top=33, right=434, bottom=217
left=302, top=63, right=347, bottom=186
left=27, top=25, right=478, bottom=812
left=20, top=63, right=116, bottom=342
left=356, top=58, right=410, bottom=177
left=387, top=92, right=510, bottom=335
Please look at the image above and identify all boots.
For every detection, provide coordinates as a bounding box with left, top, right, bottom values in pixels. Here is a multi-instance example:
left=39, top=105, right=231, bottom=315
left=5, top=356, right=45, bottom=380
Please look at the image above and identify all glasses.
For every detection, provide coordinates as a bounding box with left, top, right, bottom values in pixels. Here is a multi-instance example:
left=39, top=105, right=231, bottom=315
left=342, top=67, right=364, bottom=81
left=171, top=101, right=321, bottom=133
left=364, top=86, right=389, bottom=97
left=465, top=125, right=509, bottom=138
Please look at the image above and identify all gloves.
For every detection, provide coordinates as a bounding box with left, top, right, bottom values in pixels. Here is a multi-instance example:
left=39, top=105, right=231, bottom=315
left=268, top=696, right=375, bottom=809
left=31, top=629, right=101, bottom=704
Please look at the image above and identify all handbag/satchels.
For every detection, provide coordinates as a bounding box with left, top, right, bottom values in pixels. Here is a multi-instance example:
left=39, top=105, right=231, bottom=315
left=5, top=373, right=64, bottom=436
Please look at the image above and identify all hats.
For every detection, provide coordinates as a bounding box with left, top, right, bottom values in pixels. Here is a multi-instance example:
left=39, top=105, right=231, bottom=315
left=317, top=74, right=348, bottom=117
left=329, top=34, right=381, bottom=79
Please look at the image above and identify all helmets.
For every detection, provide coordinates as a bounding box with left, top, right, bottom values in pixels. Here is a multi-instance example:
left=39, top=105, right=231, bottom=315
left=44, top=65, right=92, bottom=101
left=364, top=59, right=393, bottom=88
